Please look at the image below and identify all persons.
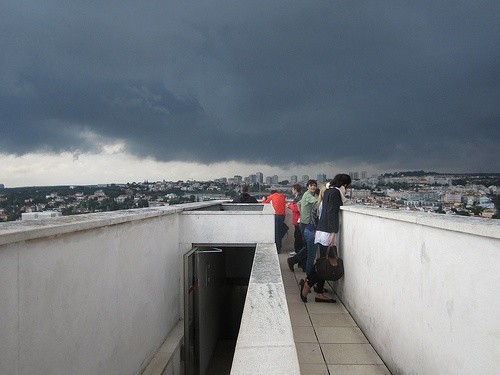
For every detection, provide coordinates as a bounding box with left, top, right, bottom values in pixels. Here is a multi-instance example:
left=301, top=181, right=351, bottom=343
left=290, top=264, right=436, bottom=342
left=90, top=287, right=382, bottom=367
left=287, top=180, right=318, bottom=272
left=289, top=184, right=303, bottom=253
left=299, top=174, right=352, bottom=303
left=232, top=185, right=257, bottom=203
left=260, top=190, right=287, bottom=254
left=304, top=185, right=327, bottom=279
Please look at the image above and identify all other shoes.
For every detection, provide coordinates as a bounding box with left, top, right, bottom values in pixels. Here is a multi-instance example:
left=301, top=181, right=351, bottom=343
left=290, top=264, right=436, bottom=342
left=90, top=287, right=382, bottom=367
left=314, top=297, right=336, bottom=303
left=300, top=282, right=307, bottom=302
left=287, top=258, right=294, bottom=272
left=314, top=286, right=327, bottom=293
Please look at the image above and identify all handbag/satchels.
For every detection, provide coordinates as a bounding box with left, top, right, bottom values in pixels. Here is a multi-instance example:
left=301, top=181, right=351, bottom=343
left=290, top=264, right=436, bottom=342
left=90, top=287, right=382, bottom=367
left=313, top=245, right=345, bottom=281
